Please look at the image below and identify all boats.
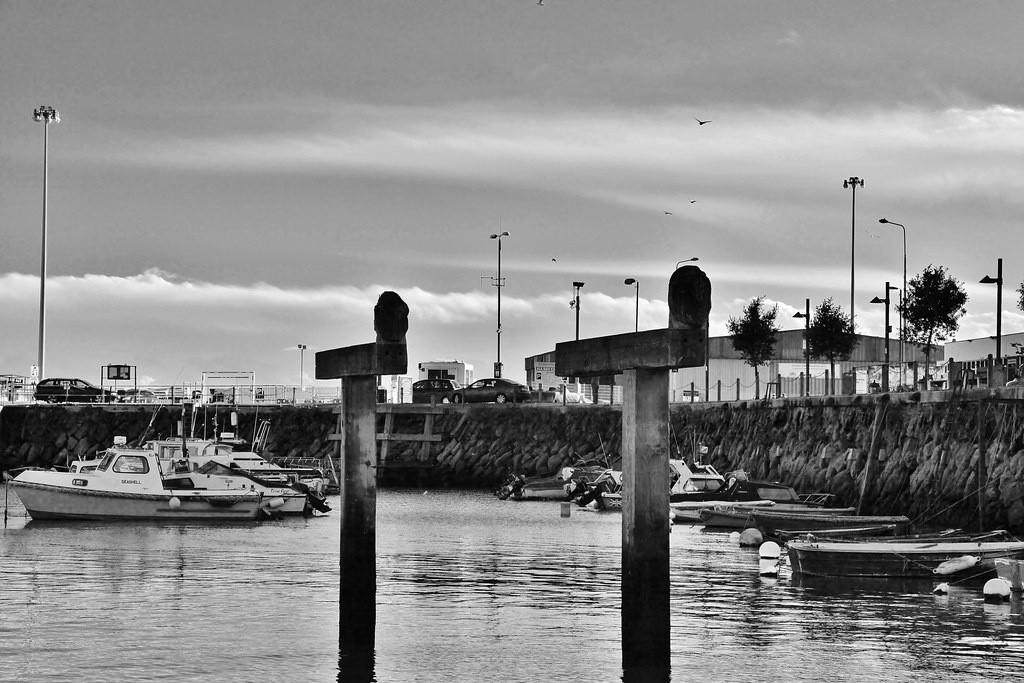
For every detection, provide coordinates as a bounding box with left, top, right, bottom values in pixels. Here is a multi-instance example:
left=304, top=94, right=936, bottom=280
left=1, top=365, right=328, bottom=521
left=698, top=503, right=854, bottom=531
left=772, top=525, right=1022, bottom=579
left=751, top=509, right=912, bottom=541
left=932, top=552, right=1024, bottom=596
left=496, top=427, right=812, bottom=525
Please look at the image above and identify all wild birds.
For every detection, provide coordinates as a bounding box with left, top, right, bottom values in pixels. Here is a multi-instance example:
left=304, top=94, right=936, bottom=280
left=552, top=258, right=557, bottom=262
left=689, top=200, right=696, bottom=203
left=694, top=117, right=712, bottom=126
left=664, top=211, right=672, bottom=215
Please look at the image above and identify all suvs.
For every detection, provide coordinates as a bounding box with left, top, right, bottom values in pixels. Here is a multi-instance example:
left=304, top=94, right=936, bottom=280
left=412, top=379, right=462, bottom=404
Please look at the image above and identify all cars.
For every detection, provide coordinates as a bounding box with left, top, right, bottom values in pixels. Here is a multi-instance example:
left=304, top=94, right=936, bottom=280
left=448, top=377, right=532, bottom=406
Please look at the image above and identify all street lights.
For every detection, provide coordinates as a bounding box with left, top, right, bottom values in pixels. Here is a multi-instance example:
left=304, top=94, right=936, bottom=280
left=879, top=219, right=909, bottom=391
left=490, top=231, right=510, bottom=376
left=980, top=258, right=1006, bottom=367
left=793, top=298, right=812, bottom=397
left=571, top=281, right=585, bottom=340
left=32, top=103, right=61, bottom=382
left=298, top=344, right=306, bottom=390
left=625, top=278, right=639, bottom=333
left=872, top=283, right=894, bottom=393
